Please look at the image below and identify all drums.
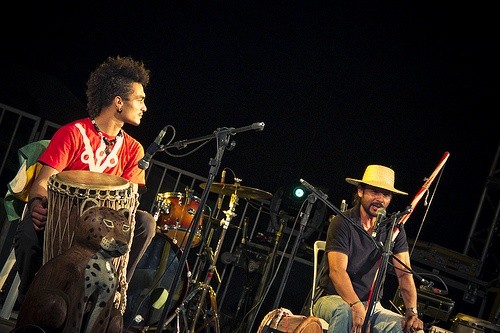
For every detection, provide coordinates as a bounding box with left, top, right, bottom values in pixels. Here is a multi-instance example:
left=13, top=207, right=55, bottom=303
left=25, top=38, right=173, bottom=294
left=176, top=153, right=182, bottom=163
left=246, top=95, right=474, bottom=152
left=43, top=171, right=139, bottom=316
left=152, top=191, right=211, bottom=251
left=124, top=229, right=191, bottom=333
left=257, top=307, right=324, bottom=333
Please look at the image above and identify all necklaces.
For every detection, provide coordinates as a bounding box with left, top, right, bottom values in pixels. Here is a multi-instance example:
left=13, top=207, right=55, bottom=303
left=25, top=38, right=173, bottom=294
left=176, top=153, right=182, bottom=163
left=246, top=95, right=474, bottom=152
left=88, top=114, right=124, bottom=155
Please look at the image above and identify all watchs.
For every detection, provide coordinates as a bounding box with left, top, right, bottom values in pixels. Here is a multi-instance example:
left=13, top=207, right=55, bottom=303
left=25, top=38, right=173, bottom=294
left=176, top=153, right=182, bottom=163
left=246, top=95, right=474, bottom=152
left=406, top=307, right=418, bottom=315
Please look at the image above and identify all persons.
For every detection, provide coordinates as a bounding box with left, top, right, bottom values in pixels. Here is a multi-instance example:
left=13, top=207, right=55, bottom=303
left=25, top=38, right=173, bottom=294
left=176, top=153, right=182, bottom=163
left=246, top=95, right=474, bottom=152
left=12, top=55, right=158, bottom=300
left=309, top=163, right=426, bottom=333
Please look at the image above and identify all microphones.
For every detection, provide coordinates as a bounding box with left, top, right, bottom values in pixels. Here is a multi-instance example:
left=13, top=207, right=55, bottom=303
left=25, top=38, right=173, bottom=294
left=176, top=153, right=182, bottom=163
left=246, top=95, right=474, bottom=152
left=138, top=128, right=168, bottom=169
left=372, top=207, right=386, bottom=238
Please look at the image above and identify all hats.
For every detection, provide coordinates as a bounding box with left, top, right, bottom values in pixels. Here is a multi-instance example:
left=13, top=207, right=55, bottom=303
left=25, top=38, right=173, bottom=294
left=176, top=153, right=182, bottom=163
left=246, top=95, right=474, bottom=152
left=346, top=163, right=409, bottom=196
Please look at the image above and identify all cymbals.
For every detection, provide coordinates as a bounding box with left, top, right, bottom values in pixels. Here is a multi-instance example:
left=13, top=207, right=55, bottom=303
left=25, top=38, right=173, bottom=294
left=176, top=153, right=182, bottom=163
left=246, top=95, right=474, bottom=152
left=196, top=181, right=273, bottom=201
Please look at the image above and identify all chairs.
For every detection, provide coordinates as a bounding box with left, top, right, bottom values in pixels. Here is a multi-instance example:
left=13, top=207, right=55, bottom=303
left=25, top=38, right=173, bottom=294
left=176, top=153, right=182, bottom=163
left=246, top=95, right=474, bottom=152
left=308, top=238, right=338, bottom=331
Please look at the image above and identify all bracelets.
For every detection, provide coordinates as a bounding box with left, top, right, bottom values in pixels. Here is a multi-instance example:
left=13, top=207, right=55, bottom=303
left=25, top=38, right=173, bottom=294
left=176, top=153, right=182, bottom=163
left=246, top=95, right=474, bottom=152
left=350, top=299, right=361, bottom=307
left=27, top=197, right=42, bottom=211
